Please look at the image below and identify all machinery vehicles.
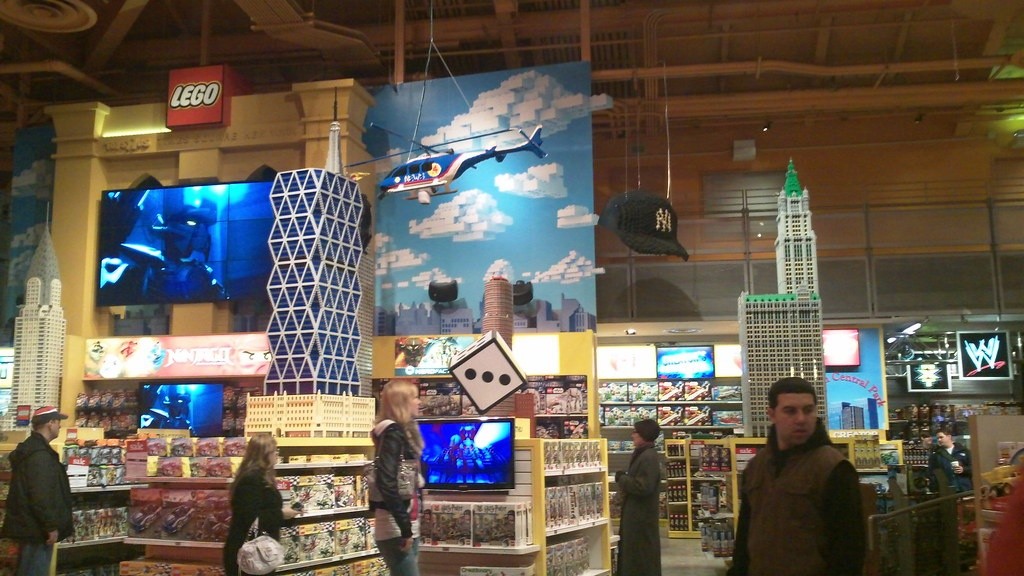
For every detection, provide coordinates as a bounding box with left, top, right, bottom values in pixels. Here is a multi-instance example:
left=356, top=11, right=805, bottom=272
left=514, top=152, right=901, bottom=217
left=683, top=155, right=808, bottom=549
left=980, top=453, right=1024, bottom=498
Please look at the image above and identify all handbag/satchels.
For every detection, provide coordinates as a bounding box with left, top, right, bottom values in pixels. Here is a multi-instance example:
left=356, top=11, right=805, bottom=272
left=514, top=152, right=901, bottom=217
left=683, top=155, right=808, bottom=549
left=368, top=432, right=418, bottom=502
left=237, top=517, right=283, bottom=574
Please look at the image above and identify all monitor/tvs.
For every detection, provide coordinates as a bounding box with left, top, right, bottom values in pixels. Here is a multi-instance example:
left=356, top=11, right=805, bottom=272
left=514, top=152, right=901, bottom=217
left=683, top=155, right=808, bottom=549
left=822, top=328, right=861, bottom=367
left=138, top=381, right=224, bottom=439
left=414, top=416, right=515, bottom=494
left=656, top=345, right=715, bottom=381
left=96, top=180, right=283, bottom=302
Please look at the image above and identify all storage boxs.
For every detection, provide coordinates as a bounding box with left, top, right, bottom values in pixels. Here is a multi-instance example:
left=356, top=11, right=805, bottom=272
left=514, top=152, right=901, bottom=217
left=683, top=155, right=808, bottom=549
left=0, top=375, right=758, bottom=575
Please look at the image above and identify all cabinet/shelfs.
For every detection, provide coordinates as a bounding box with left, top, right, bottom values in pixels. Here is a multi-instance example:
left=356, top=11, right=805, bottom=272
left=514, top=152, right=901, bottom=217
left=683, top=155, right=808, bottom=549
left=409, top=437, right=618, bottom=576
left=0, top=428, right=141, bottom=567
left=112, top=425, right=378, bottom=576
left=363, top=328, right=601, bottom=435
left=602, top=337, right=974, bottom=544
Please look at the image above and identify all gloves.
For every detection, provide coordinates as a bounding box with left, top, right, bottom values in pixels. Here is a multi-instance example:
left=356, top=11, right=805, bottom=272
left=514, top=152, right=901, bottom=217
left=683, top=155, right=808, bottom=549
left=615, top=471, right=625, bottom=482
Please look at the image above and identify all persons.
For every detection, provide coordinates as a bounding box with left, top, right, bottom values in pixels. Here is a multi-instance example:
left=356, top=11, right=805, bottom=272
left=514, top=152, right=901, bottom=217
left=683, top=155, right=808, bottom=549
left=614, top=419, right=662, bottom=576
left=929, top=428, right=971, bottom=491
left=726, top=376, right=865, bottom=576
left=920, top=432, right=933, bottom=444
left=1, top=406, right=76, bottom=576
left=223, top=433, right=301, bottom=576
left=368, top=379, right=428, bottom=576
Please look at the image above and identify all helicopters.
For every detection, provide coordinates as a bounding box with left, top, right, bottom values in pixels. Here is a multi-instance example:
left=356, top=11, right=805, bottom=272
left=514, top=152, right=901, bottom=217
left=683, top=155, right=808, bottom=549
left=342, top=122, right=550, bottom=206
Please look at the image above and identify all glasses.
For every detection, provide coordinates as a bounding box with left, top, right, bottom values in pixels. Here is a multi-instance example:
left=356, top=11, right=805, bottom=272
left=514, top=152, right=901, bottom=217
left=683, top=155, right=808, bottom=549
left=632, top=430, right=638, bottom=435
left=273, top=448, right=280, bottom=454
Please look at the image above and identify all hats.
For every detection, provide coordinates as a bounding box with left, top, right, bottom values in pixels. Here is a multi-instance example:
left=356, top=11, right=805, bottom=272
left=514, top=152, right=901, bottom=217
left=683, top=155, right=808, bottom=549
left=31, top=406, right=68, bottom=423
left=599, top=190, right=690, bottom=262
left=634, top=419, right=660, bottom=442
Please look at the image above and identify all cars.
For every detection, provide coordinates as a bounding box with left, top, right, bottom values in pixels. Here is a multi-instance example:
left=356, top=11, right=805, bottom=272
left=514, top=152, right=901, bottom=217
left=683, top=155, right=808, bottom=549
left=161, top=504, right=197, bottom=535
left=128, top=502, right=163, bottom=532
